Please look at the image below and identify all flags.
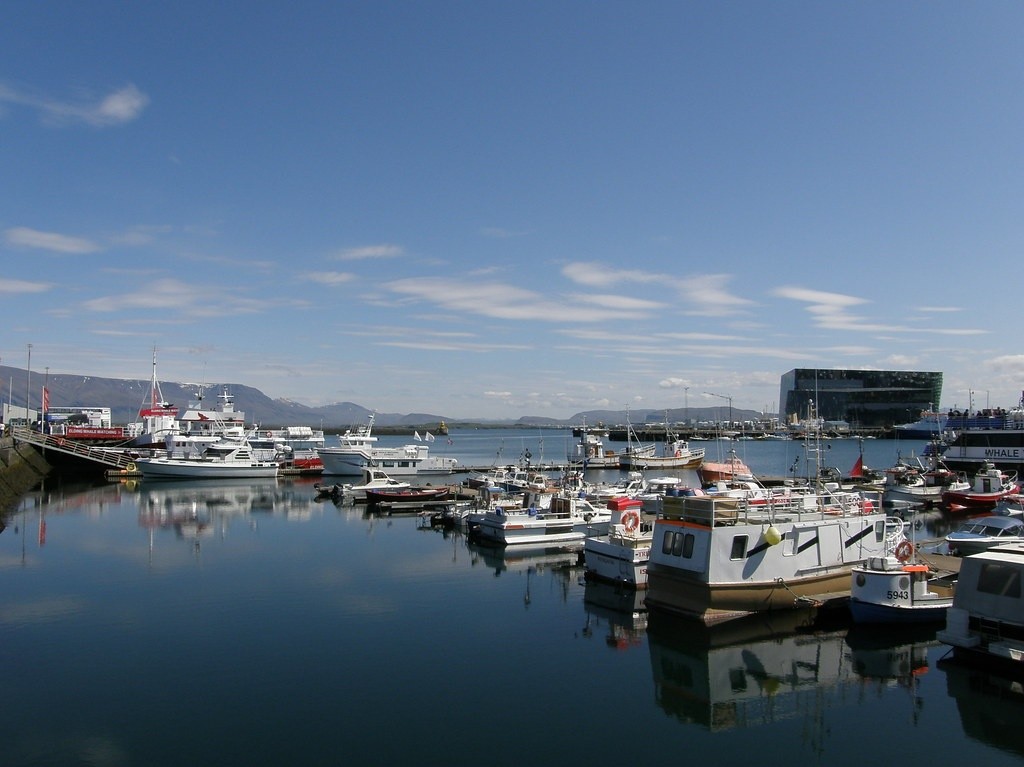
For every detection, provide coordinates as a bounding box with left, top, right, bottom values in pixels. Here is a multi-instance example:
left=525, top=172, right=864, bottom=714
left=447, top=438, right=455, bottom=446
left=413, top=430, right=422, bottom=442
left=43, top=387, right=49, bottom=413
left=849, top=454, right=862, bottom=478
left=424, top=431, right=435, bottom=443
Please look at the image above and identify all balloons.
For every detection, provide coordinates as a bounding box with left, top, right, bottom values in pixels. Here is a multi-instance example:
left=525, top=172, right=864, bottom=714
left=764, top=674, right=780, bottom=695
left=765, top=526, right=781, bottom=545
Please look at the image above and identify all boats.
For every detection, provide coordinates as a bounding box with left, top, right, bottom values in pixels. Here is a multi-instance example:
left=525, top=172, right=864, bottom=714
left=128, top=342, right=458, bottom=476
left=313, top=389, right=1023, bottom=663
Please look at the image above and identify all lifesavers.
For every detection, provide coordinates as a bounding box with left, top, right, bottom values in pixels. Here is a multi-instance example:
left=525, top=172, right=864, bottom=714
left=895, top=541, right=913, bottom=561
left=622, top=512, right=639, bottom=532
left=266, top=430, right=272, bottom=438
left=823, top=507, right=844, bottom=515
left=57, top=437, right=66, bottom=446
left=675, top=450, right=681, bottom=457
left=126, top=462, right=137, bottom=472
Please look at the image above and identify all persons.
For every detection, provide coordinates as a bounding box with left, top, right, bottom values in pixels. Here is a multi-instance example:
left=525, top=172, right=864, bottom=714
left=948, top=406, right=1024, bottom=431
left=47, top=419, right=146, bottom=437
left=524, top=448, right=532, bottom=466
left=0, top=421, right=5, bottom=438
